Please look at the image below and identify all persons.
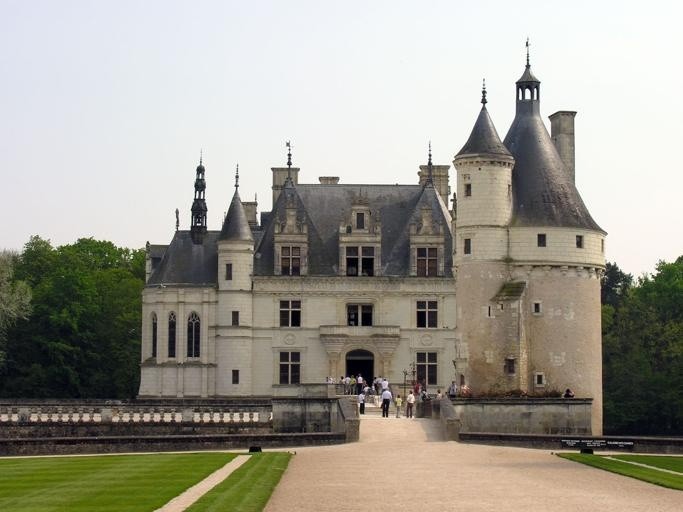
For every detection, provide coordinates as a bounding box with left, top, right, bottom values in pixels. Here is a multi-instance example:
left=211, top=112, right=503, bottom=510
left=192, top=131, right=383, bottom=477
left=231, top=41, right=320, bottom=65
left=340, top=373, right=458, bottom=419
